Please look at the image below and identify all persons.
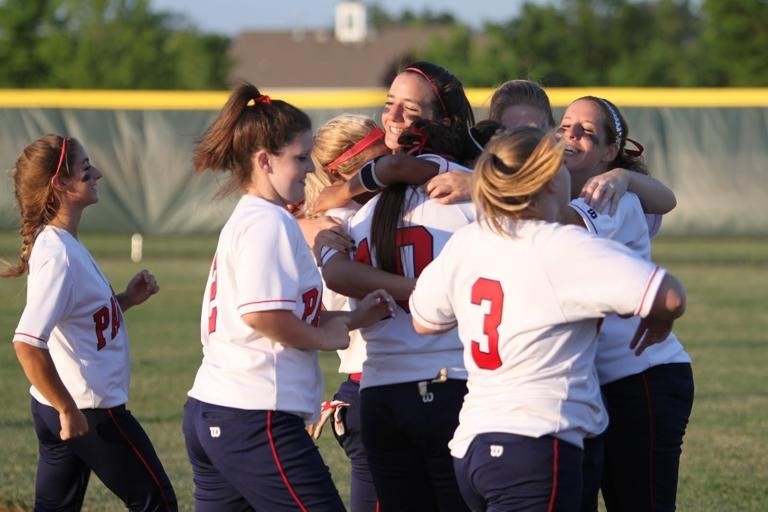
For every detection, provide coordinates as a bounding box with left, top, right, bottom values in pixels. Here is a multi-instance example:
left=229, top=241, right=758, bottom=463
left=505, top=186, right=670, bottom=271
left=0, top=132, right=185, bottom=512
left=184, top=79, right=351, bottom=511
left=294, top=60, right=698, bottom=512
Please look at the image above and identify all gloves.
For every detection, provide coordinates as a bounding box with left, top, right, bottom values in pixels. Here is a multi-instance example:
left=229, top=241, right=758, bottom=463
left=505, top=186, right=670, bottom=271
left=306, top=400, right=351, bottom=439
